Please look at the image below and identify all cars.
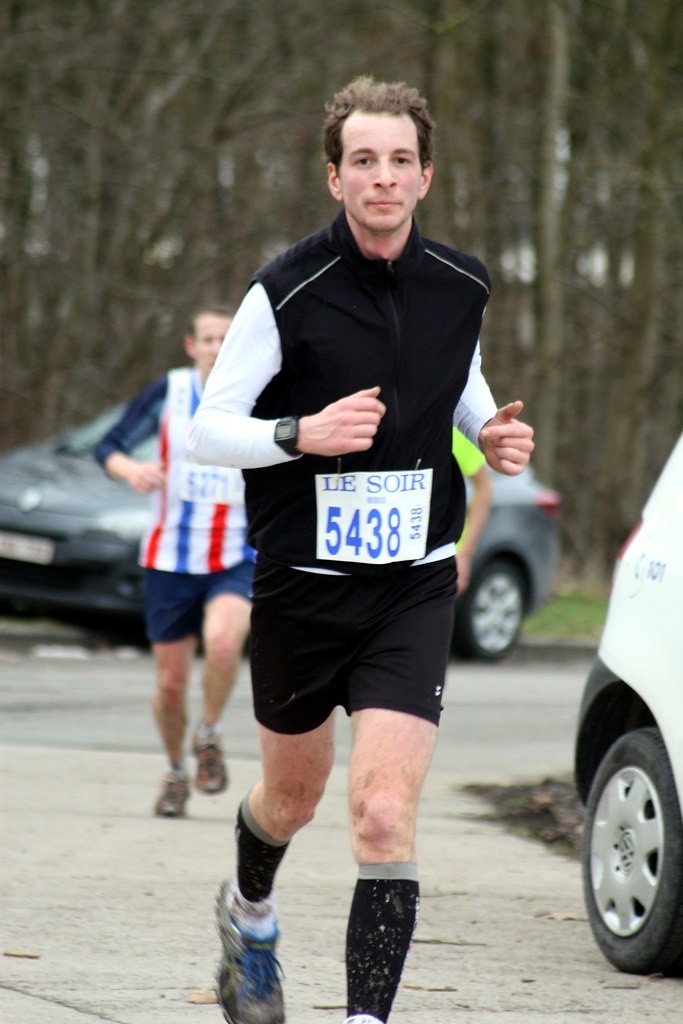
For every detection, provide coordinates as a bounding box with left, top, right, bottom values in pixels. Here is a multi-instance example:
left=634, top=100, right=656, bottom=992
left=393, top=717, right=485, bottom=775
left=0, top=397, right=564, bottom=664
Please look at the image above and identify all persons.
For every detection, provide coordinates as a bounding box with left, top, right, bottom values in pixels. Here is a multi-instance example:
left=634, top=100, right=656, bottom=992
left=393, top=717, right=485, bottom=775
left=452, top=425, right=494, bottom=603
left=183, top=75, right=532, bottom=1024
left=91, top=304, right=257, bottom=815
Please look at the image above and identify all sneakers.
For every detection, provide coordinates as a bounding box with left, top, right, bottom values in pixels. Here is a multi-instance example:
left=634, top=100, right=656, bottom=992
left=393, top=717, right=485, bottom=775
left=156, top=772, right=191, bottom=815
left=215, top=876, right=286, bottom=1024
left=191, top=729, right=229, bottom=796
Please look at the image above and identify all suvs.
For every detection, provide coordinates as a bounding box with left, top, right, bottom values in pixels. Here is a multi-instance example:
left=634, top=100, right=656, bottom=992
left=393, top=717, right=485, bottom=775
left=574, top=437, right=683, bottom=977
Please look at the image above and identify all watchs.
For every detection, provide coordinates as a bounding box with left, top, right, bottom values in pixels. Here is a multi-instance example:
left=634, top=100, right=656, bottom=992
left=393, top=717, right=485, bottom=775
left=274, top=416, right=303, bottom=456
